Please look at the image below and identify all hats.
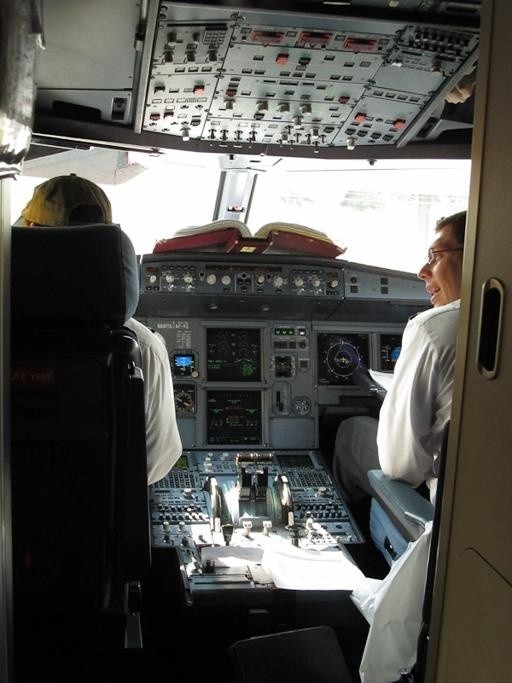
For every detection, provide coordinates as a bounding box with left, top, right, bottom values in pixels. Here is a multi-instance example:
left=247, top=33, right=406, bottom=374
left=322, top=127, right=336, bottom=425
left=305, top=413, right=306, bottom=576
left=21, top=174, right=112, bottom=226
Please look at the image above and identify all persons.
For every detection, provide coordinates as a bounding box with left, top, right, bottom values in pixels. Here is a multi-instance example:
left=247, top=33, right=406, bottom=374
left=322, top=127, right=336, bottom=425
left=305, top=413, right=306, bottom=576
left=21, top=169, right=182, bottom=484
left=331, top=208, right=467, bottom=509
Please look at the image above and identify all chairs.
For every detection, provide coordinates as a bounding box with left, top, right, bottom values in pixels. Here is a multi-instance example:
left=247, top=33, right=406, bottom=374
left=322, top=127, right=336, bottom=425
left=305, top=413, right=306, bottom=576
left=10, top=224, right=149, bottom=676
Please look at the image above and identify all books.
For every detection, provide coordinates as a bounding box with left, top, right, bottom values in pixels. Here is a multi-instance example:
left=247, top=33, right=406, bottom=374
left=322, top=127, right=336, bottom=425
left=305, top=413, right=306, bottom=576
left=151, top=219, right=341, bottom=261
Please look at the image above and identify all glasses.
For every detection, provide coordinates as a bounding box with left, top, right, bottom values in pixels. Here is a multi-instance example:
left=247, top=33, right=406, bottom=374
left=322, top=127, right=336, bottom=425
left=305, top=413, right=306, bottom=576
left=425, top=248, right=463, bottom=264
